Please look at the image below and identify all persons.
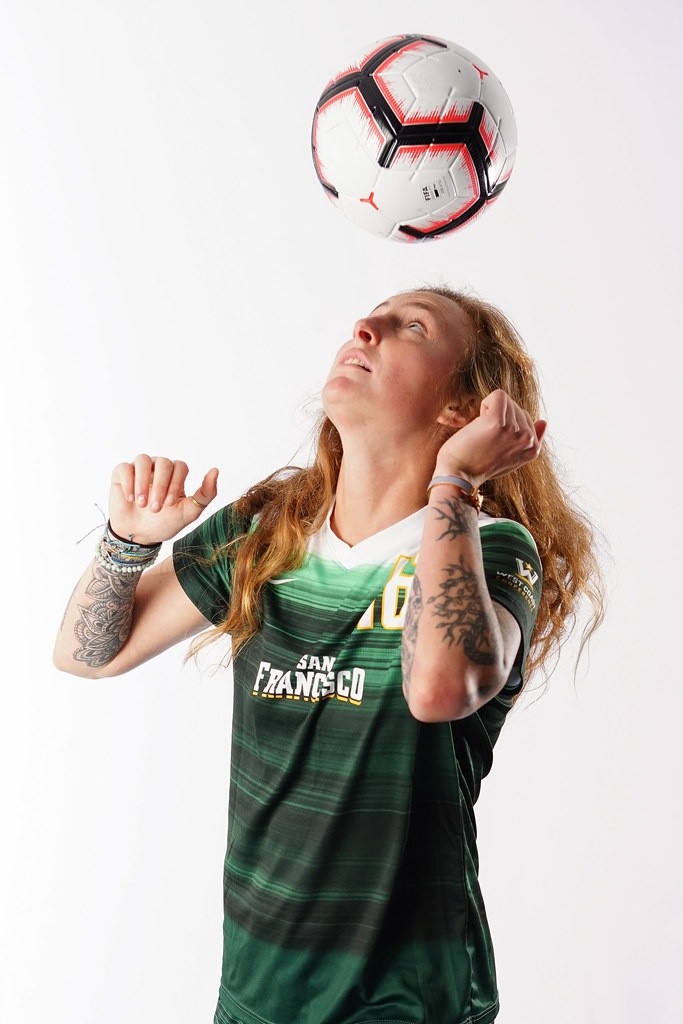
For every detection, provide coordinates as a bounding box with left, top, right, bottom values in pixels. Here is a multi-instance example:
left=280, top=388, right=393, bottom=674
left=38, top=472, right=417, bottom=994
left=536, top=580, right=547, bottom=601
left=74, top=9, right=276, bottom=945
left=53, top=275, right=610, bottom=1024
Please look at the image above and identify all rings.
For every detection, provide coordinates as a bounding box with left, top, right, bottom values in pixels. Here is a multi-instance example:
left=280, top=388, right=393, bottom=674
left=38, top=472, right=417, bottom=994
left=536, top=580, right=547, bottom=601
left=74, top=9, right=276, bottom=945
left=191, top=496, right=207, bottom=509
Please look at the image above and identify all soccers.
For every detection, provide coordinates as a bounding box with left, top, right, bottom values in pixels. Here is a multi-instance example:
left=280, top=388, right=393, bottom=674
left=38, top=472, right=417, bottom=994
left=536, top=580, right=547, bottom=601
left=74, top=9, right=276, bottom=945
left=308, top=26, right=520, bottom=246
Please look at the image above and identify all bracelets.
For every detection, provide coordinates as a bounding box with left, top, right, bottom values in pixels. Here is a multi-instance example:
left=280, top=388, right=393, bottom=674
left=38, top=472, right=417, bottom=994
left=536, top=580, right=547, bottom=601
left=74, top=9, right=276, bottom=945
left=76, top=502, right=163, bottom=573
left=427, top=474, right=483, bottom=516
